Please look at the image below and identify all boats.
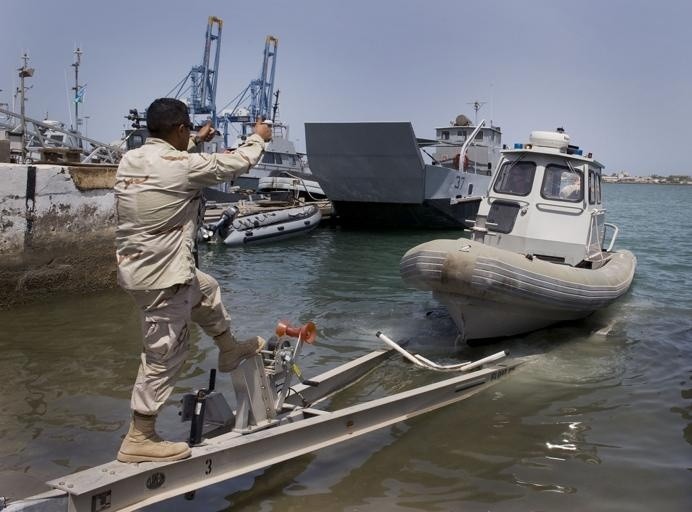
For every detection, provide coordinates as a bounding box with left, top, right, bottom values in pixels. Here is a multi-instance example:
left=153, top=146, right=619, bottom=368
left=398, top=124, right=639, bottom=342
left=303, top=98, right=513, bottom=227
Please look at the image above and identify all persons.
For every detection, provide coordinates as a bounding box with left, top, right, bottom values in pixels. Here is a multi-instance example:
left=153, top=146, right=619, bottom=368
left=507, top=167, right=528, bottom=194
left=110, top=95, right=268, bottom=465
left=566, top=178, right=580, bottom=200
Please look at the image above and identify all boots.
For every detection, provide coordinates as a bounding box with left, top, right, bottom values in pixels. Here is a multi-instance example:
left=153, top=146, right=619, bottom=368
left=212, top=327, right=266, bottom=374
left=117, top=408, right=193, bottom=464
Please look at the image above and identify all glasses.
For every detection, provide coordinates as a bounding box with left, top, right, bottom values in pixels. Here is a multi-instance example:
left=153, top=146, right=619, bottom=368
left=184, top=122, right=194, bottom=131
